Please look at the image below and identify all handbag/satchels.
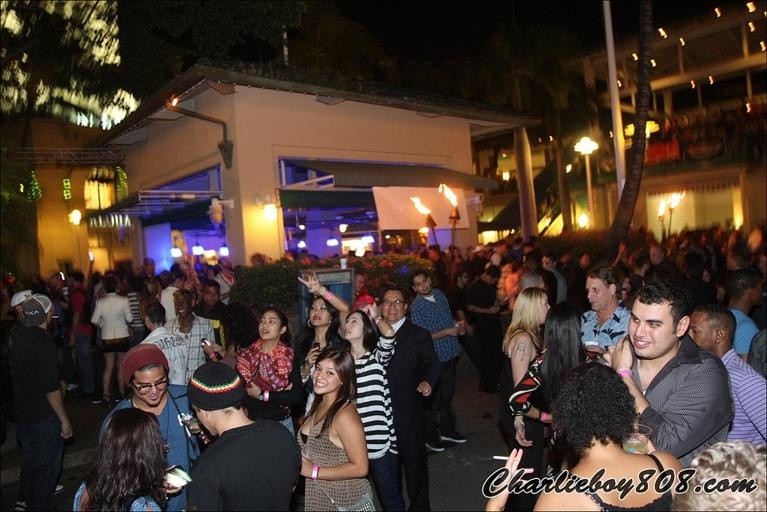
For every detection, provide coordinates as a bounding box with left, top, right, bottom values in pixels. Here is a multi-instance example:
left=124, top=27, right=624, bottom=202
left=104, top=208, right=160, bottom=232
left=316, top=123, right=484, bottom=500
left=338, top=493, right=376, bottom=511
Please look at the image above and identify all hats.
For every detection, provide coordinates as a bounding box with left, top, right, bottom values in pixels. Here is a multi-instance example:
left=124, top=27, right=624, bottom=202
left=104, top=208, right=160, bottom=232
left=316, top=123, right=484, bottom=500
left=21, top=293, right=53, bottom=327
left=10, top=289, right=35, bottom=309
left=121, top=344, right=169, bottom=387
left=187, top=361, right=244, bottom=409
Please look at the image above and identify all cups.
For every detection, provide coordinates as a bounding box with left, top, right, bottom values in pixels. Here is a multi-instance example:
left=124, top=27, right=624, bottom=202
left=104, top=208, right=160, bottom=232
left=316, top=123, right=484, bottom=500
left=455, top=320, right=465, bottom=335
left=622, top=423, right=652, bottom=455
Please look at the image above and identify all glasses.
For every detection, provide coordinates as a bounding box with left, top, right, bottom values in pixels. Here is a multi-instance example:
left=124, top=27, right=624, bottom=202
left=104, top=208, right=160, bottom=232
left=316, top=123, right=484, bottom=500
left=129, top=377, right=169, bottom=395
left=381, top=298, right=407, bottom=306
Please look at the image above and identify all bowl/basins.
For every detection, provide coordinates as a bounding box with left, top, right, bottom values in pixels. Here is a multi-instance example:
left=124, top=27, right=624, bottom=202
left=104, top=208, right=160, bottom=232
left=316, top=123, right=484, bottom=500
left=585, top=341, right=600, bottom=349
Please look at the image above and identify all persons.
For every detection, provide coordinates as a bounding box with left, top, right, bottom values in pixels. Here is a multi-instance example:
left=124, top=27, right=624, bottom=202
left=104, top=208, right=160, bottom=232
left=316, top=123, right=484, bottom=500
left=1, top=217, right=766, bottom=512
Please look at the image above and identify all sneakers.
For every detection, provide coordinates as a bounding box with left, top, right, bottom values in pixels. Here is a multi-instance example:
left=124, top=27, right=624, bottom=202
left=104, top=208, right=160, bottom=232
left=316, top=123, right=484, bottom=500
left=440, top=430, right=468, bottom=443
left=49, top=484, right=65, bottom=496
left=13, top=498, right=28, bottom=511
left=424, top=438, right=445, bottom=452
left=66, top=382, right=126, bottom=407
